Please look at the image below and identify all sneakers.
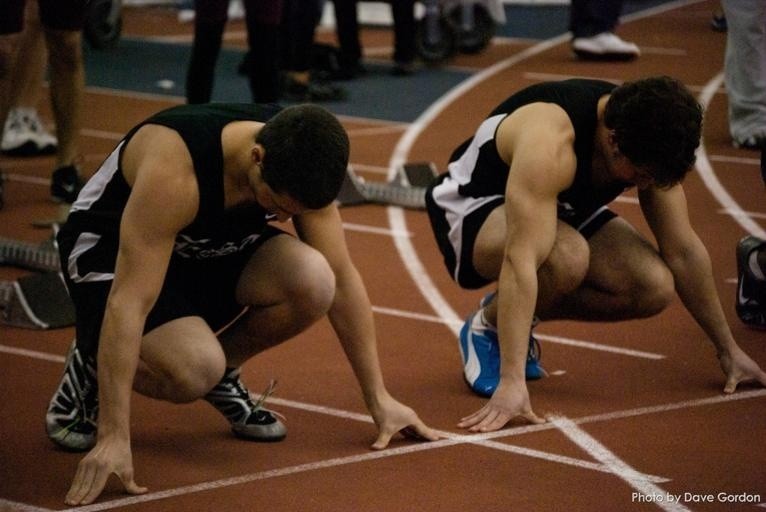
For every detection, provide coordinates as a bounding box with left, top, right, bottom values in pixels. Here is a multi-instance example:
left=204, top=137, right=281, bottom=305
left=736, top=236, right=765, bottom=329
left=572, top=32, right=641, bottom=58
left=280, top=70, right=345, bottom=102
left=202, top=368, right=286, bottom=442
left=1, top=107, right=58, bottom=156
left=46, top=337, right=99, bottom=448
left=458, top=292, right=543, bottom=393
left=50, top=165, right=87, bottom=202
left=734, top=131, right=765, bottom=150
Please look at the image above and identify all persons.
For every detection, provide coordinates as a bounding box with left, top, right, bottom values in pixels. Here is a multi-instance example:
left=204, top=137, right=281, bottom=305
left=422, top=73, right=765, bottom=435
left=732, top=236, right=765, bottom=334
left=43, top=101, right=442, bottom=510
left=2, top=1, right=766, bottom=233
